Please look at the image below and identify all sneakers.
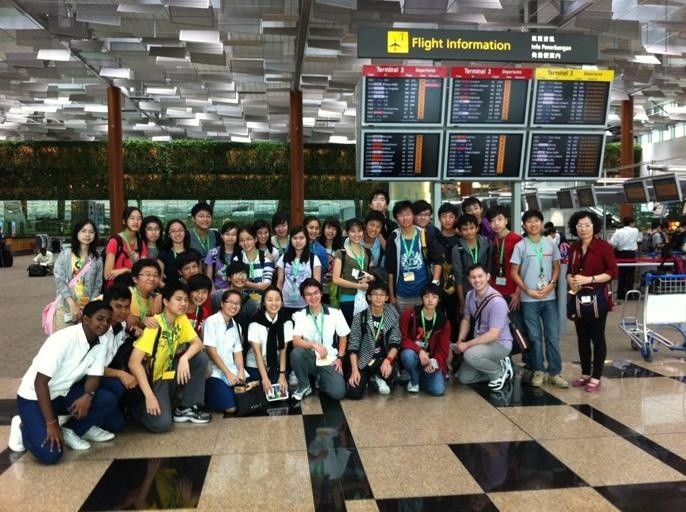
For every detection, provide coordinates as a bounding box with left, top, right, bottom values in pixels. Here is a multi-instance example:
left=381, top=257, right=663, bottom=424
left=288, top=370, right=321, bottom=401
left=586, top=377, right=601, bottom=391
left=488, top=356, right=569, bottom=390
left=81, top=425, right=116, bottom=442
left=573, top=375, right=591, bottom=386
left=60, top=423, right=91, bottom=450
left=369, top=369, right=420, bottom=394
left=8, top=414, right=26, bottom=452
left=173, top=407, right=212, bottom=423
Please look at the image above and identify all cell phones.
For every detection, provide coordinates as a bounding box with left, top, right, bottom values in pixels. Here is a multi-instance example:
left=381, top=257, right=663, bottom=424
left=358, top=271, right=366, bottom=280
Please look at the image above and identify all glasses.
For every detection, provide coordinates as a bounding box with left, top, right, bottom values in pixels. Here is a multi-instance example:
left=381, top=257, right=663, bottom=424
left=144, top=227, right=160, bottom=232
left=138, top=271, right=159, bottom=278
left=576, top=223, right=593, bottom=230
left=224, top=300, right=240, bottom=307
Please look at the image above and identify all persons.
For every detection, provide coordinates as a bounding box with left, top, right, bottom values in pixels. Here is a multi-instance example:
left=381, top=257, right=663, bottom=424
left=7, top=192, right=569, bottom=464
left=565, top=210, right=618, bottom=393
left=478, top=442, right=509, bottom=492
left=306, top=412, right=351, bottom=496
left=607, top=216, right=643, bottom=301
left=650, top=221, right=669, bottom=258
left=118, top=458, right=191, bottom=511
left=669, top=224, right=686, bottom=252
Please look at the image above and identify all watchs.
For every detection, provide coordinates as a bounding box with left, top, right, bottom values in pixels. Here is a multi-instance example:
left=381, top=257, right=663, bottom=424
left=592, top=276, right=596, bottom=284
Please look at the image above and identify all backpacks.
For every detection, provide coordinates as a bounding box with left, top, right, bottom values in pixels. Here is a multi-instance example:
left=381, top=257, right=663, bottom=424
left=100, top=231, right=142, bottom=294
left=642, top=231, right=660, bottom=252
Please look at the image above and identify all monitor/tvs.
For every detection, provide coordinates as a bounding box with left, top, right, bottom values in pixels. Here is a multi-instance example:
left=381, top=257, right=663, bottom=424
left=443, top=129, right=527, bottom=181
left=447, top=77, right=532, bottom=128
left=557, top=191, right=574, bottom=209
left=524, top=130, right=606, bottom=180
left=576, top=187, right=597, bottom=207
left=361, top=75, right=447, bottom=128
left=651, top=173, right=682, bottom=203
left=530, top=79, right=612, bottom=129
left=360, top=129, right=443, bottom=181
left=623, top=180, right=650, bottom=203
left=521, top=192, right=541, bottom=212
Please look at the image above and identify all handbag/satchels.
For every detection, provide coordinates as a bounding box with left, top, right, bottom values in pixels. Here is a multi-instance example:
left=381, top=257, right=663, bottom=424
left=233, top=378, right=270, bottom=416
left=29, top=264, right=47, bottom=276
left=428, top=257, right=456, bottom=295
left=641, top=269, right=673, bottom=286
left=673, top=255, right=686, bottom=280
left=567, top=287, right=607, bottom=319
left=41, top=301, right=56, bottom=336
left=329, top=281, right=340, bottom=308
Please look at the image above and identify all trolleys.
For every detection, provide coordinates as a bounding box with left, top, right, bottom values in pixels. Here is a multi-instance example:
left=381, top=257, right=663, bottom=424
left=613, top=271, right=685, bottom=359
